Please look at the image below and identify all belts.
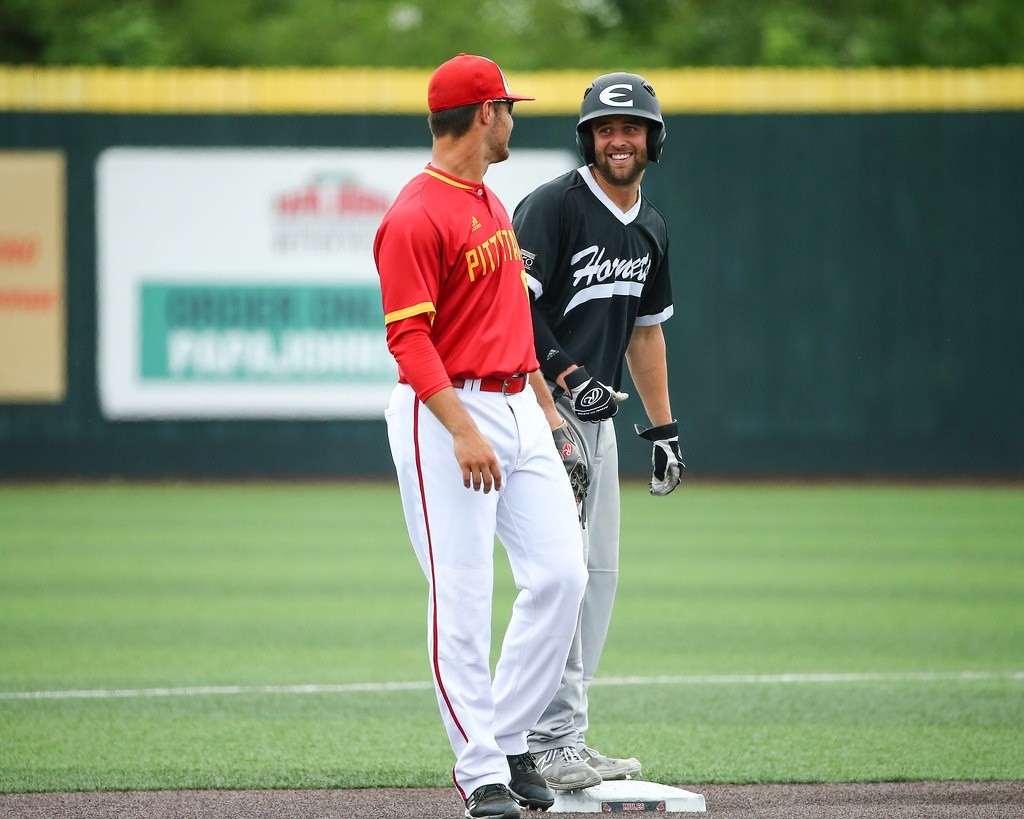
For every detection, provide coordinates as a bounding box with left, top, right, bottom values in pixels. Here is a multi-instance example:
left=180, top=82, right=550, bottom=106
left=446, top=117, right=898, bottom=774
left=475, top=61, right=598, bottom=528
left=399, top=371, right=527, bottom=396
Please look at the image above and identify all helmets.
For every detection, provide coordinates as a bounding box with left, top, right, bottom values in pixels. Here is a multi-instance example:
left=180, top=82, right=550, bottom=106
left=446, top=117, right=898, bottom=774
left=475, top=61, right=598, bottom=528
left=576, top=72, right=666, bottom=167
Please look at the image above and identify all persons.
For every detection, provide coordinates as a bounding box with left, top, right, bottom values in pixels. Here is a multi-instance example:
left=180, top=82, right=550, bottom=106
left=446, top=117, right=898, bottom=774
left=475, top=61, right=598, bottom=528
left=509, top=74, right=687, bottom=794
left=373, top=50, right=593, bottom=819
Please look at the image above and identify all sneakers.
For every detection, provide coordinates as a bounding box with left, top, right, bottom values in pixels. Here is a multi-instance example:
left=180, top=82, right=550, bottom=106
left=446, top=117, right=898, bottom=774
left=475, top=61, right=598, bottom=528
left=465, top=784, right=521, bottom=819
left=504, top=751, right=555, bottom=813
left=578, top=747, right=641, bottom=780
left=529, top=746, right=602, bottom=794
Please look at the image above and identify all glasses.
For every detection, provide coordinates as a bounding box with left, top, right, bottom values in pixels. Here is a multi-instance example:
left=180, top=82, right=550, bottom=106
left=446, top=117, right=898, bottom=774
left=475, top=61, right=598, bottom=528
left=486, top=99, right=513, bottom=115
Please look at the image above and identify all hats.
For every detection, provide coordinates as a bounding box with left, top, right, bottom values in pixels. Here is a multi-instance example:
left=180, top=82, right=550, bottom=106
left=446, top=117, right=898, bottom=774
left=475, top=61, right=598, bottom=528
left=428, top=53, right=535, bottom=113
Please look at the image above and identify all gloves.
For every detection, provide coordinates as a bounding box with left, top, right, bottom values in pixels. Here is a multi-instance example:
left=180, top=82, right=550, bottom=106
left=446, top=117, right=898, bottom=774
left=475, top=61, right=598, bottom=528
left=634, top=419, right=685, bottom=496
left=552, top=366, right=630, bottom=424
